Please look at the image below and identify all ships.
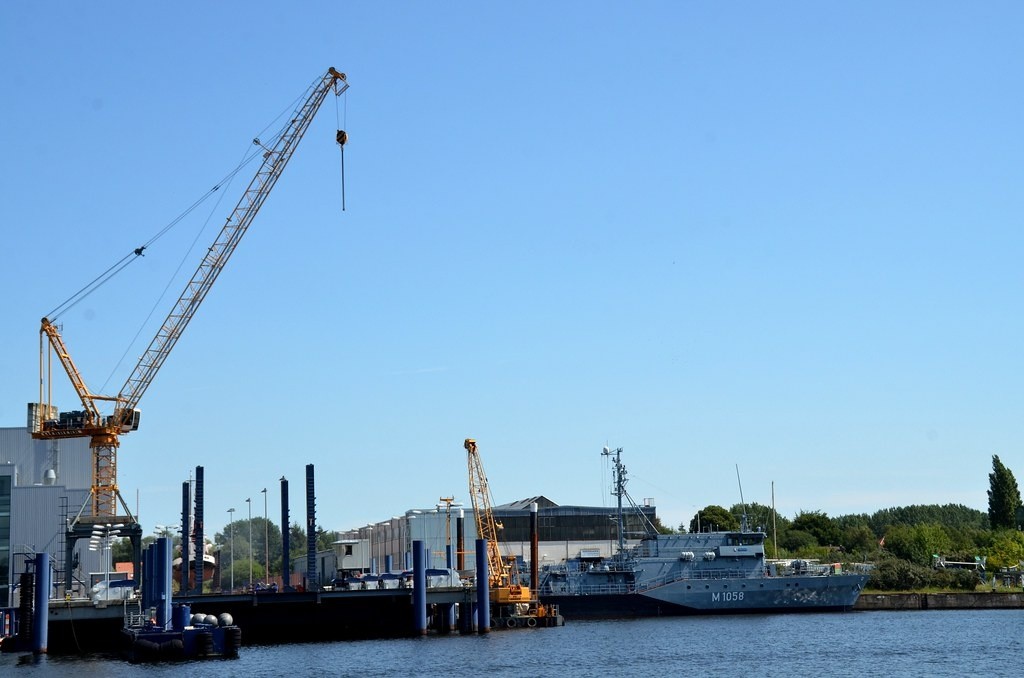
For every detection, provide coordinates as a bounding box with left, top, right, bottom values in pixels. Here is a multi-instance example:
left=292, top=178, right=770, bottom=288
left=510, top=441, right=871, bottom=614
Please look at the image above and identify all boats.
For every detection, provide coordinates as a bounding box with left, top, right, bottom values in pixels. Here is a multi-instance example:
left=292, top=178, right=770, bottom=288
left=119, top=597, right=242, bottom=663
left=170, top=469, right=215, bottom=585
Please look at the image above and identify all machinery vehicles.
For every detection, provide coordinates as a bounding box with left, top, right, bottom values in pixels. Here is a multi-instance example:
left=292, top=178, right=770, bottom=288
left=464, top=438, right=540, bottom=616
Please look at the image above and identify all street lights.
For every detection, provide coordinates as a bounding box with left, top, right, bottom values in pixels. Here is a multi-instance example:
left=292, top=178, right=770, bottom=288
left=245, top=498, right=252, bottom=591
left=412, top=509, right=438, bottom=569
left=367, top=522, right=390, bottom=574
left=93, top=523, right=124, bottom=601
left=436, top=502, right=464, bottom=588
left=393, top=516, right=416, bottom=571
left=353, top=527, right=373, bottom=574
left=262, top=488, right=269, bottom=585
left=156, top=524, right=177, bottom=629
left=227, top=508, right=235, bottom=595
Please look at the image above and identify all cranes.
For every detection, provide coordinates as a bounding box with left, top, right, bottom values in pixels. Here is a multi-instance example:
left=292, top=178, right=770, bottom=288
left=25, top=65, right=352, bottom=597
